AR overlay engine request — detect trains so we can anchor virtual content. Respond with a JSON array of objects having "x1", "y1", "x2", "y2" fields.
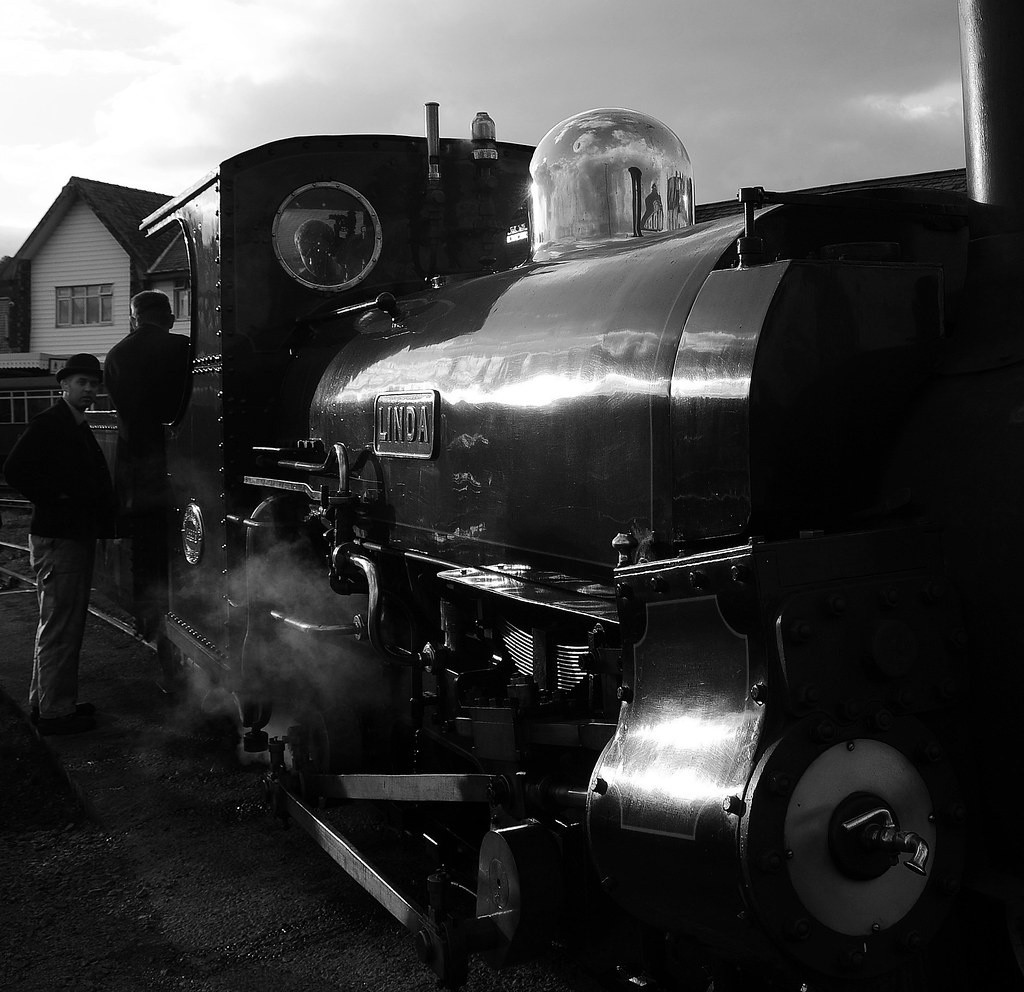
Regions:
[{"x1": 78, "y1": 102, "x2": 1024, "y2": 992}]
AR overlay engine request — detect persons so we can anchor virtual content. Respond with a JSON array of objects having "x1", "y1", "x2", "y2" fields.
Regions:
[
  {"x1": 0, "y1": 353, "x2": 119, "y2": 731},
  {"x1": 102, "y1": 290, "x2": 192, "y2": 641}
]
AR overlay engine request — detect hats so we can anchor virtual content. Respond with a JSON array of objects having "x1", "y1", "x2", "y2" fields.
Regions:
[{"x1": 56, "y1": 354, "x2": 104, "y2": 382}]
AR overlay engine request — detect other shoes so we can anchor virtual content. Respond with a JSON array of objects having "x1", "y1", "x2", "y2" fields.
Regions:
[{"x1": 31, "y1": 704, "x2": 96, "y2": 736}]
